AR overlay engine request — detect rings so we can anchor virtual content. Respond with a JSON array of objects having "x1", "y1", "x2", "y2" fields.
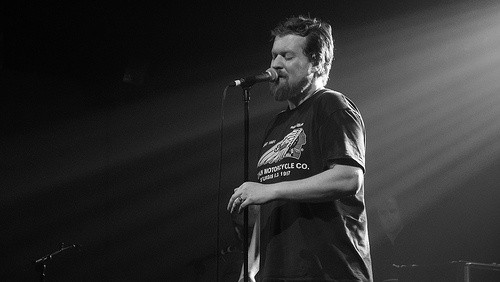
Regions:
[{"x1": 238, "y1": 196, "x2": 244, "y2": 204}]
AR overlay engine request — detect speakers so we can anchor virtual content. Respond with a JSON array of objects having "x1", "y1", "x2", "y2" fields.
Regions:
[{"x1": 456, "y1": 260, "x2": 500, "y2": 282}]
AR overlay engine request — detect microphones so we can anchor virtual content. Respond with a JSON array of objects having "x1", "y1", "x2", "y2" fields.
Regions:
[{"x1": 228, "y1": 67, "x2": 279, "y2": 88}]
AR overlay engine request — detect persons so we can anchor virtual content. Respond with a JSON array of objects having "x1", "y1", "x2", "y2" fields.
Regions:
[{"x1": 225, "y1": 15, "x2": 373, "y2": 282}]
